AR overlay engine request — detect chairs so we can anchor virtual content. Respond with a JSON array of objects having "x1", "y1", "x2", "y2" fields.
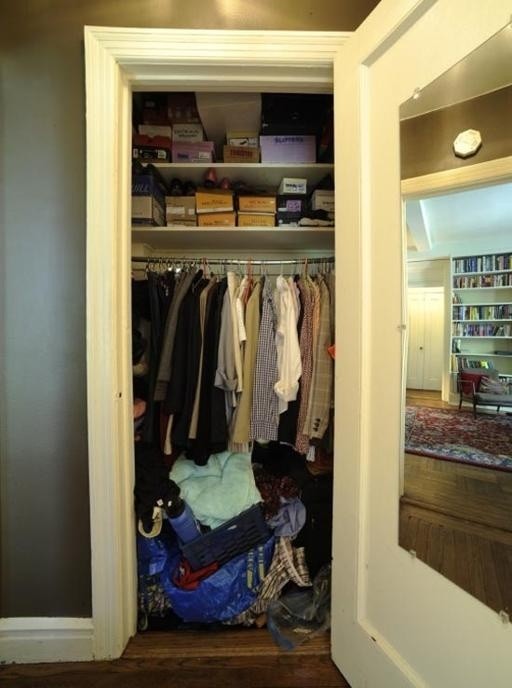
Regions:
[{"x1": 455, "y1": 367, "x2": 509, "y2": 418}]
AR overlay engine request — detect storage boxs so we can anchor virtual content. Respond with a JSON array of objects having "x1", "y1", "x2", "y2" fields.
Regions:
[{"x1": 131, "y1": 118, "x2": 335, "y2": 228}]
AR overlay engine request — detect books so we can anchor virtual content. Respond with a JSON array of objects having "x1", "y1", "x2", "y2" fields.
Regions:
[{"x1": 451, "y1": 256, "x2": 512, "y2": 371}]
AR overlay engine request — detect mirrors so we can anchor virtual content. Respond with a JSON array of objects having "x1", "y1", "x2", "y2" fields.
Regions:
[{"x1": 396, "y1": 151, "x2": 511, "y2": 626}]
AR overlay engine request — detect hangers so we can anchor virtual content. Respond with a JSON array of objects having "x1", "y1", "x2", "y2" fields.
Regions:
[{"x1": 132, "y1": 253, "x2": 334, "y2": 290}]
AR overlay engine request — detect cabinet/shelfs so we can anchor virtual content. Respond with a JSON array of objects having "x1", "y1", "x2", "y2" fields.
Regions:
[
  {"x1": 125, "y1": 84, "x2": 336, "y2": 247},
  {"x1": 444, "y1": 250, "x2": 511, "y2": 426}
]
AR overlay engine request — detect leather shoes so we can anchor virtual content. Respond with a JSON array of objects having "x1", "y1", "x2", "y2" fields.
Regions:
[{"x1": 132, "y1": 161, "x2": 249, "y2": 196}]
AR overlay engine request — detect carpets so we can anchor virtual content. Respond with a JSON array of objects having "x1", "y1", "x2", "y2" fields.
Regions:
[{"x1": 405, "y1": 402, "x2": 511, "y2": 471}]
